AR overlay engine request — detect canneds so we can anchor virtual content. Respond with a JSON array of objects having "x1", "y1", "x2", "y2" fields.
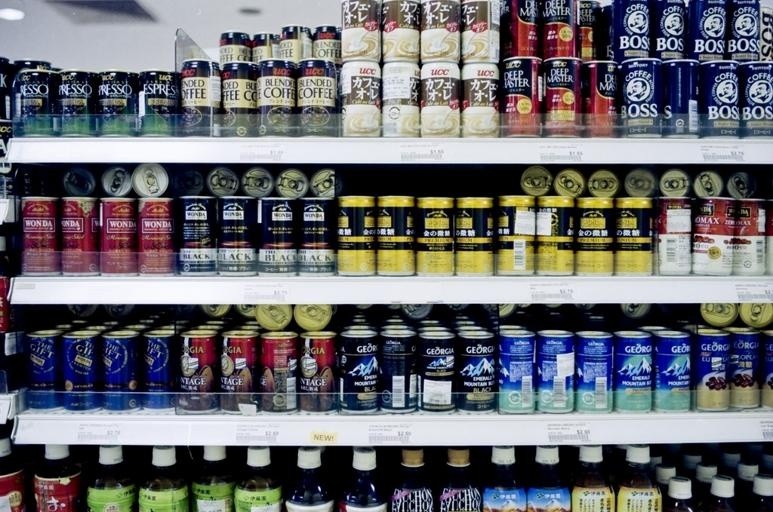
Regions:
[
  {"x1": 2, "y1": 303, "x2": 772, "y2": 418},
  {"x1": 0, "y1": 0, "x2": 772, "y2": 137},
  {"x1": 2, "y1": 165, "x2": 772, "y2": 275}
]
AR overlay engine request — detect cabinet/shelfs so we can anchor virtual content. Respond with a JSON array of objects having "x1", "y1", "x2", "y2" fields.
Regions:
[{"x1": 3, "y1": 25, "x2": 773, "y2": 455}]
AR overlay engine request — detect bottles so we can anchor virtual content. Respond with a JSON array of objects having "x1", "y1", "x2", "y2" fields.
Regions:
[
  {"x1": 0, "y1": 435, "x2": 773, "y2": 511},
  {"x1": 0, "y1": 236, "x2": 11, "y2": 334}
]
